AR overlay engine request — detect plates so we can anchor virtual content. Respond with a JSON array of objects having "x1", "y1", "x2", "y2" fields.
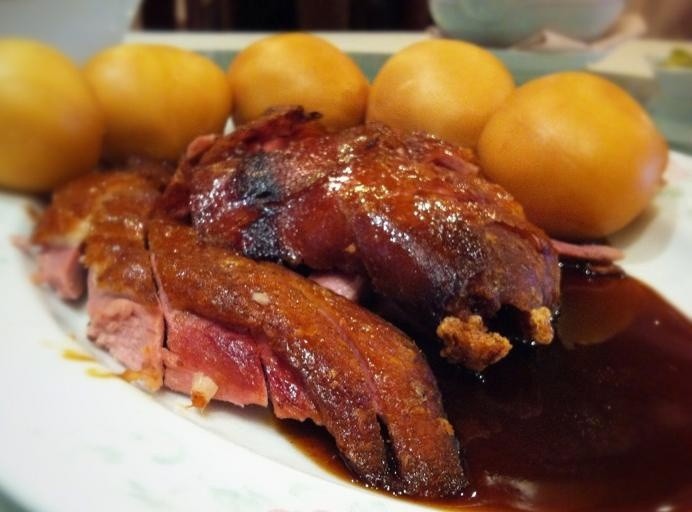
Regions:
[
  {"x1": 645, "y1": 103, "x2": 692, "y2": 146},
  {"x1": 0, "y1": 149, "x2": 692, "y2": 512},
  {"x1": 422, "y1": 30, "x2": 619, "y2": 85}
]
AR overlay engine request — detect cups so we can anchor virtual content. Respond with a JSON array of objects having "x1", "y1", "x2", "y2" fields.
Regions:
[
  {"x1": 0, "y1": 0, "x2": 139, "y2": 70},
  {"x1": 653, "y1": 69, "x2": 692, "y2": 125},
  {"x1": 428, "y1": 0, "x2": 621, "y2": 48}
]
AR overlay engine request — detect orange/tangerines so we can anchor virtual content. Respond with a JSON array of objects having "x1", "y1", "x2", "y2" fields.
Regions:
[
  {"x1": 476, "y1": 72, "x2": 669, "y2": 237},
  {"x1": 365, "y1": 39, "x2": 515, "y2": 149},
  {"x1": 226, "y1": 33, "x2": 371, "y2": 135},
  {"x1": 79, "y1": 43, "x2": 233, "y2": 166},
  {"x1": 0, "y1": 37, "x2": 106, "y2": 195}
]
